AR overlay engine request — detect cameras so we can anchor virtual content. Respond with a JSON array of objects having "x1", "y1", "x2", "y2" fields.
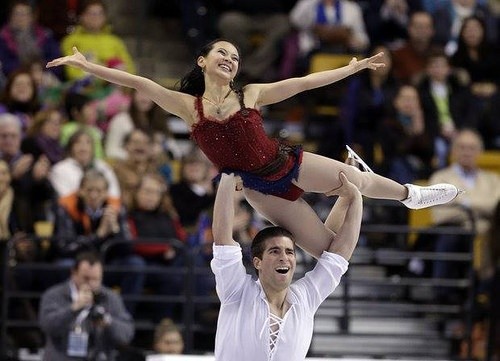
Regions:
[{"x1": 93, "y1": 292, "x2": 107, "y2": 305}]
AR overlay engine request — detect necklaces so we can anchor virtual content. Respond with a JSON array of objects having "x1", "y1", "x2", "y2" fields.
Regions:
[{"x1": 202, "y1": 90, "x2": 231, "y2": 115}]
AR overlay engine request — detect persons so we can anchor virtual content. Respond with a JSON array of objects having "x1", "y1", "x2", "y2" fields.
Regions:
[
  {"x1": 0, "y1": 0, "x2": 264, "y2": 361},
  {"x1": 219, "y1": 0, "x2": 500, "y2": 361},
  {"x1": 211, "y1": 172, "x2": 363, "y2": 361},
  {"x1": 46, "y1": 39, "x2": 458, "y2": 260}
]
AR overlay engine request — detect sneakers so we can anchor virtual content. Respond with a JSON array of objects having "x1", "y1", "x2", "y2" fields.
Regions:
[{"x1": 402, "y1": 183, "x2": 464, "y2": 209}]
